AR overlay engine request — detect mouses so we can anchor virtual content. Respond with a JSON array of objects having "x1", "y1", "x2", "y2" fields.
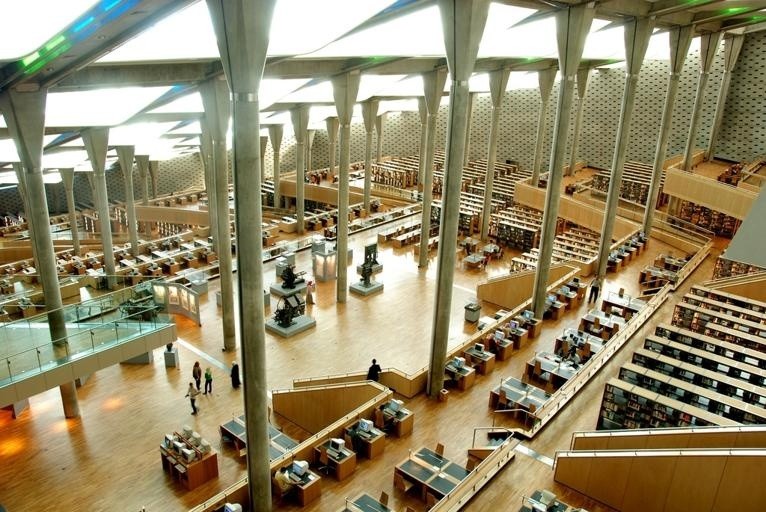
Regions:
[{"x1": 456, "y1": 370, "x2": 459, "y2": 372}]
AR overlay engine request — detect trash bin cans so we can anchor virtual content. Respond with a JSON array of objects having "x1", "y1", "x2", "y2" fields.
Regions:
[
  {"x1": 440, "y1": 389, "x2": 449, "y2": 402},
  {"x1": 222, "y1": 434, "x2": 234, "y2": 449},
  {"x1": 464, "y1": 303, "x2": 482, "y2": 323}
]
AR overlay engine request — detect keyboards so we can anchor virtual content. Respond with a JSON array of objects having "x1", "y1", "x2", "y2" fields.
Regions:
[
  {"x1": 174, "y1": 464, "x2": 186, "y2": 472},
  {"x1": 447, "y1": 365, "x2": 457, "y2": 371},
  {"x1": 290, "y1": 473, "x2": 302, "y2": 482}
]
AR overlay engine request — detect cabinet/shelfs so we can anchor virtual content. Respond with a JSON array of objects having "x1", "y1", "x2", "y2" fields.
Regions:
[
  {"x1": 517, "y1": 284, "x2": 766, "y2": 511},
  {"x1": 3, "y1": 188, "x2": 211, "y2": 239},
  {"x1": 227, "y1": 166, "x2": 333, "y2": 212},
  {"x1": 261, "y1": 241, "x2": 384, "y2": 339},
  {"x1": 75, "y1": 347, "x2": 180, "y2": 388},
  {"x1": 370, "y1": 149, "x2": 766, "y2": 282}
]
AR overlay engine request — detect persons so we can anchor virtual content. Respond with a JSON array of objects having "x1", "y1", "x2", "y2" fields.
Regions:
[
  {"x1": 366, "y1": 358, "x2": 381, "y2": 382},
  {"x1": 3, "y1": 214, "x2": 26, "y2": 227},
  {"x1": 465, "y1": 243, "x2": 470, "y2": 256},
  {"x1": 561, "y1": 349, "x2": 582, "y2": 366},
  {"x1": 192, "y1": 361, "x2": 202, "y2": 390},
  {"x1": 202, "y1": 367, "x2": 212, "y2": 394},
  {"x1": 184, "y1": 382, "x2": 201, "y2": 415},
  {"x1": 305, "y1": 169, "x2": 328, "y2": 186},
  {"x1": 587, "y1": 275, "x2": 602, "y2": 304},
  {"x1": 229, "y1": 360, "x2": 241, "y2": 390}
]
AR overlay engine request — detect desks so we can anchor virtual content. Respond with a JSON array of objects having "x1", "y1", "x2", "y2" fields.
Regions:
[
  {"x1": 1, "y1": 227, "x2": 236, "y2": 322},
  {"x1": 159, "y1": 428, "x2": 219, "y2": 491},
  {"x1": 220, "y1": 320, "x2": 554, "y2": 509},
  {"x1": 250, "y1": 196, "x2": 370, "y2": 260}
]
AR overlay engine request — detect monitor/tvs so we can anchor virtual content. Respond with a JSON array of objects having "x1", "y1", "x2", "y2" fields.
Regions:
[
  {"x1": 292, "y1": 398, "x2": 404, "y2": 475},
  {"x1": 452, "y1": 233, "x2": 645, "y2": 369},
  {"x1": 540, "y1": 489, "x2": 557, "y2": 509},
  {"x1": 224, "y1": 502, "x2": 242, "y2": 512},
  {"x1": 165, "y1": 425, "x2": 211, "y2": 464}
]
